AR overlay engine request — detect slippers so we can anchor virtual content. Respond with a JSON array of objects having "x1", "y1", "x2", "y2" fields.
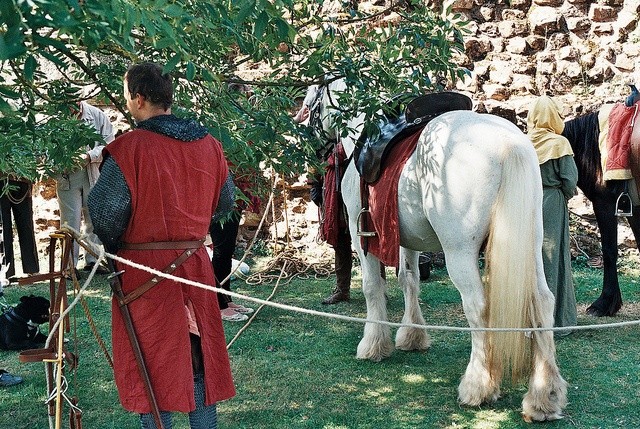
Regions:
[
  {"x1": 221, "y1": 308, "x2": 250, "y2": 322},
  {"x1": 235, "y1": 304, "x2": 255, "y2": 315}
]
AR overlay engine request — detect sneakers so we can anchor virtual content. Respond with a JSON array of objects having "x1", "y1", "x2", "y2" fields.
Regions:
[
  {"x1": 61, "y1": 268, "x2": 82, "y2": 281},
  {"x1": 324, "y1": 290, "x2": 351, "y2": 304},
  {"x1": 85, "y1": 262, "x2": 110, "y2": 274},
  {"x1": 385, "y1": 293, "x2": 390, "y2": 303}
]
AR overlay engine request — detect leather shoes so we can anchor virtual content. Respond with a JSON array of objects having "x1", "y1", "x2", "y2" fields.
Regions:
[
  {"x1": 0, "y1": 370, "x2": 24, "y2": 387},
  {"x1": 28, "y1": 272, "x2": 42, "y2": 277}
]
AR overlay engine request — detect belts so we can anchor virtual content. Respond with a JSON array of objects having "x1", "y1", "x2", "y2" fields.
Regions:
[
  {"x1": 114, "y1": 239, "x2": 205, "y2": 308},
  {"x1": 123, "y1": 239, "x2": 202, "y2": 250}
]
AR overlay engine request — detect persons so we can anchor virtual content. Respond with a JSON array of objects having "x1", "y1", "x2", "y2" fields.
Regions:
[
  {"x1": 210, "y1": 76, "x2": 309, "y2": 323},
  {"x1": 0, "y1": 92, "x2": 42, "y2": 281},
  {"x1": 54, "y1": 89, "x2": 114, "y2": 278},
  {"x1": 523, "y1": 95, "x2": 579, "y2": 336},
  {"x1": 310, "y1": 69, "x2": 379, "y2": 306},
  {"x1": 87, "y1": 61, "x2": 240, "y2": 429}
]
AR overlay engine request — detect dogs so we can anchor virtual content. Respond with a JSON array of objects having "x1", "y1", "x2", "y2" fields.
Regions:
[{"x1": 0, "y1": 293, "x2": 51, "y2": 350}]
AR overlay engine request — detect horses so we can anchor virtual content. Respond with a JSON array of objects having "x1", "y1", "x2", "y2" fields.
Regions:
[
  {"x1": 560, "y1": 109, "x2": 640, "y2": 316},
  {"x1": 304, "y1": 69, "x2": 571, "y2": 422}
]
